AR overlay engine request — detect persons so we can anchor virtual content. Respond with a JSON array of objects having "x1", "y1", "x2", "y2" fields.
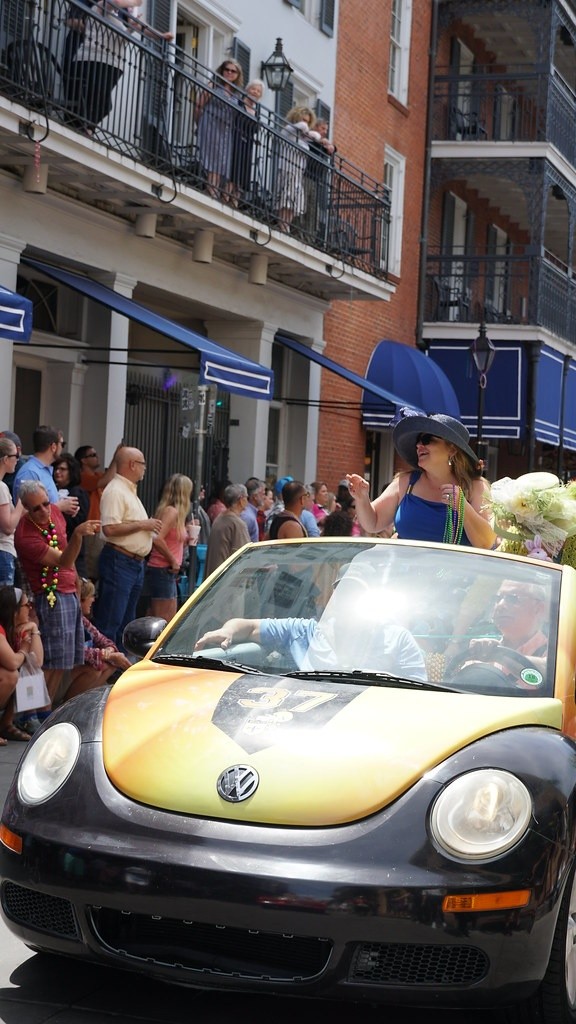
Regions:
[
  {"x1": 460, "y1": 578, "x2": 549, "y2": 689},
  {"x1": 271, "y1": 105, "x2": 337, "y2": 241},
  {"x1": 192, "y1": 59, "x2": 245, "y2": 201},
  {"x1": 0, "y1": 422, "x2": 162, "y2": 747},
  {"x1": 479, "y1": 470, "x2": 576, "y2": 570},
  {"x1": 61, "y1": 0, "x2": 174, "y2": 136},
  {"x1": 346, "y1": 414, "x2": 498, "y2": 574},
  {"x1": 194, "y1": 561, "x2": 429, "y2": 683},
  {"x1": 144, "y1": 468, "x2": 398, "y2": 634},
  {"x1": 221, "y1": 79, "x2": 263, "y2": 208}
]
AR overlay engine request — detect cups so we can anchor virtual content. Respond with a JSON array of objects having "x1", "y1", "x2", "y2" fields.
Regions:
[{"x1": 187, "y1": 525, "x2": 201, "y2": 546}]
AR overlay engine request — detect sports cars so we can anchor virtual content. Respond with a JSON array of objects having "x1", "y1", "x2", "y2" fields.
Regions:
[{"x1": 0, "y1": 536, "x2": 576, "y2": 1024}]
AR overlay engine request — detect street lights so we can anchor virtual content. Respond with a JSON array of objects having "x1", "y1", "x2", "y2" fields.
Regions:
[{"x1": 467, "y1": 325, "x2": 496, "y2": 460}]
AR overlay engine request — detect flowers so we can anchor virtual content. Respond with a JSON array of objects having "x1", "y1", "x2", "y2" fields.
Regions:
[{"x1": 478, "y1": 478, "x2": 576, "y2": 542}]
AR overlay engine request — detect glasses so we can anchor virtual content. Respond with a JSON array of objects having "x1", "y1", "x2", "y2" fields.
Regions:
[
  {"x1": 83, "y1": 453, "x2": 97, "y2": 458},
  {"x1": 81, "y1": 577, "x2": 88, "y2": 586},
  {"x1": 26, "y1": 501, "x2": 51, "y2": 513},
  {"x1": 20, "y1": 602, "x2": 31, "y2": 609},
  {"x1": 50, "y1": 439, "x2": 66, "y2": 448},
  {"x1": 223, "y1": 67, "x2": 238, "y2": 73},
  {"x1": 0, "y1": 452, "x2": 20, "y2": 460},
  {"x1": 200, "y1": 486, "x2": 205, "y2": 492},
  {"x1": 300, "y1": 493, "x2": 310, "y2": 502},
  {"x1": 415, "y1": 434, "x2": 440, "y2": 447},
  {"x1": 350, "y1": 505, "x2": 355, "y2": 509},
  {"x1": 54, "y1": 467, "x2": 69, "y2": 472},
  {"x1": 495, "y1": 593, "x2": 540, "y2": 607},
  {"x1": 131, "y1": 459, "x2": 147, "y2": 466}
]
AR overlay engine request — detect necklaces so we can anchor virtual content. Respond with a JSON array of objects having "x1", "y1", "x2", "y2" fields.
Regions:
[
  {"x1": 442, "y1": 484, "x2": 466, "y2": 544},
  {"x1": 27, "y1": 513, "x2": 59, "y2": 608},
  {"x1": 426, "y1": 652, "x2": 446, "y2": 683}
]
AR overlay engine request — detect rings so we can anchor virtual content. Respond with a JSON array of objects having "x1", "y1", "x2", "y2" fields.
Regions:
[{"x1": 446, "y1": 495, "x2": 449, "y2": 500}]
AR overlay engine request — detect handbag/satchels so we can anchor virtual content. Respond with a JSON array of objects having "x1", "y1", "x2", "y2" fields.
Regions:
[{"x1": 13, "y1": 651, "x2": 46, "y2": 713}]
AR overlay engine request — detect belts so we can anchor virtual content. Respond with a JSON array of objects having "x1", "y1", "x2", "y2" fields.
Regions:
[{"x1": 107, "y1": 541, "x2": 145, "y2": 563}]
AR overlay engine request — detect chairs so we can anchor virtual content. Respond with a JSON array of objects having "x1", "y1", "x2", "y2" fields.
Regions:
[
  {"x1": 149, "y1": 124, "x2": 207, "y2": 192},
  {"x1": 487, "y1": 304, "x2": 530, "y2": 326},
  {"x1": 451, "y1": 104, "x2": 488, "y2": 141},
  {"x1": 7, "y1": 39, "x2": 87, "y2": 132},
  {"x1": 239, "y1": 181, "x2": 376, "y2": 274},
  {"x1": 432, "y1": 277, "x2": 471, "y2": 322}
]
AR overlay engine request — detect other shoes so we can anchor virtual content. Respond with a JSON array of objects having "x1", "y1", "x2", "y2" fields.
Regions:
[
  {"x1": 26, "y1": 718, "x2": 42, "y2": 734},
  {"x1": 0, "y1": 722, "x2": 31, "y2": 745}
]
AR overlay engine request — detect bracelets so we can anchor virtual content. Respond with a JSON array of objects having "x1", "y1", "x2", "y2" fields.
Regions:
[{"x1": 31, "y1": 631, "x2": 41, "y2": 635}]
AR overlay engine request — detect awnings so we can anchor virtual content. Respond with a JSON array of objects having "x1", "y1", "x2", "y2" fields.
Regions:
[
  {"x1": 0, "y1": 284, "x2": 33, "y2": 344},
  {"x1": 274, "y1": 334, "x2": 426, "y2": 429},
  {"x1": 13, "y1": 255, "x2": 275, "y2": 401},
  {"x1": 361, "y1": 338, "x2": 461, "y2": 429}
]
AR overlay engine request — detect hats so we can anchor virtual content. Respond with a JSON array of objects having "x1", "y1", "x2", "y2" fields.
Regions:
[
  {"x1": 392, "y1": 413, "x2": 484, "y2": 478},
  {"x1": 332, "y1": 561, "x2": 375, "y2": 591},
  {"x1": 491, "y1": 471, "x2": 576, "y2": 546},
  {"x1": 274, "y1": 476, "x2": 293, "y2": 494}
]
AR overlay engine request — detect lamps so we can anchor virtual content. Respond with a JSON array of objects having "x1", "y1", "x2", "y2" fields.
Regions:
[{"x1": 260, "y1": 37, "x2": 294, "y2": 91}]
